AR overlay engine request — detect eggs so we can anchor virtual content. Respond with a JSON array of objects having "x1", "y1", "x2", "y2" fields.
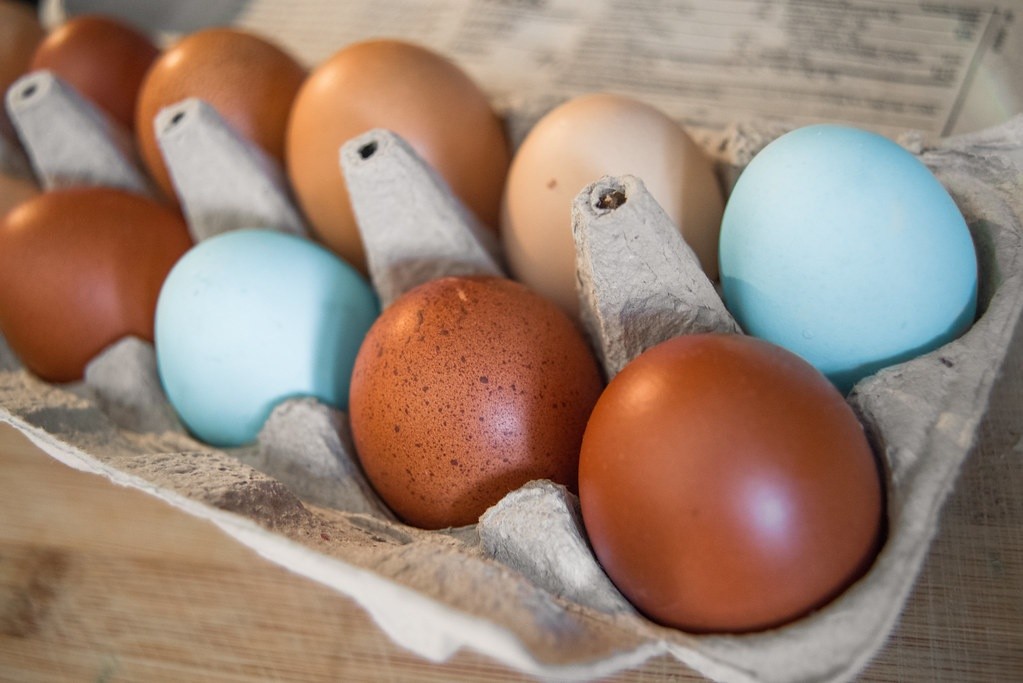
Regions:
[{"x1": 0, "y1": 7, "x2": 976, "y2": 634}]
